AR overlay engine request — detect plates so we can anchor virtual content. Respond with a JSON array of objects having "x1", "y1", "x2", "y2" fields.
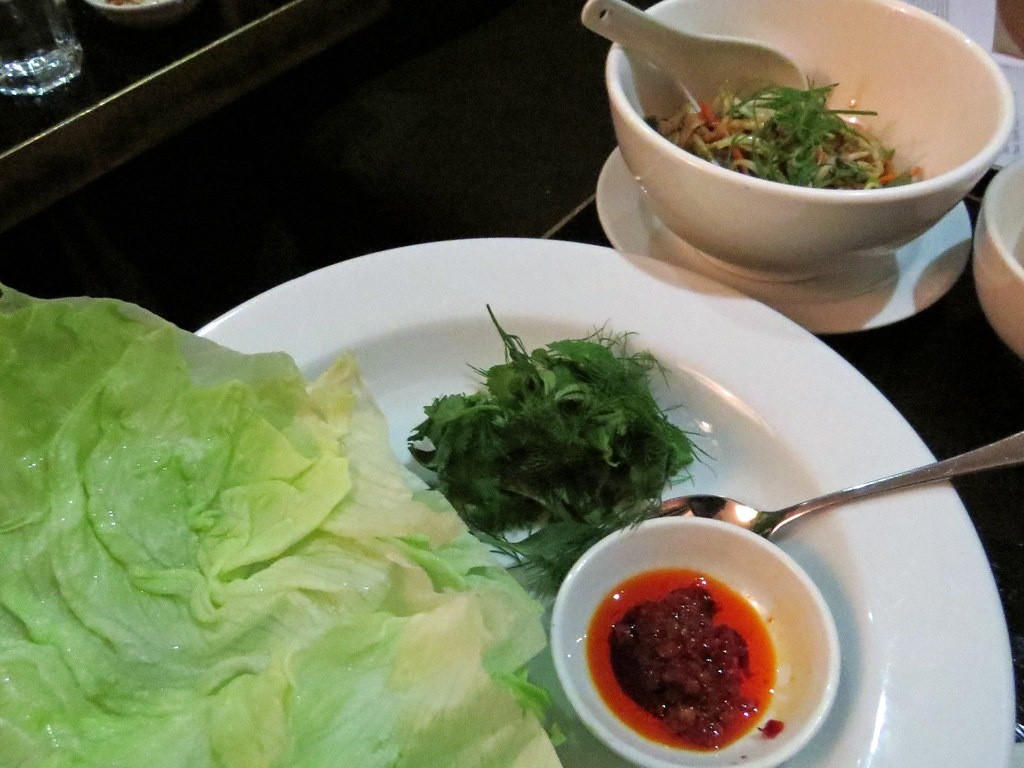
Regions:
[
  {"x1": 595, "y1": 145, "x2": 974, "y2": 333},
  {"x1": 195, "y1": 236, "x2": 1015, "y2": 768}
]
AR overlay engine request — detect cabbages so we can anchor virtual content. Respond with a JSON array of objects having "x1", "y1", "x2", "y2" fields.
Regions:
[{"x1": 0, "y1": 284, "x2": 578, "y2": 768}]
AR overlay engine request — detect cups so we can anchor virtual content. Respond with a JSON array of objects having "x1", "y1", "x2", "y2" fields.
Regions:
[{"x1": 0, "y1": 0, "x2": 84, "y2": 98}]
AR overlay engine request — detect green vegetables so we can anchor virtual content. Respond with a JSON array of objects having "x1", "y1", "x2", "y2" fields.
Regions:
[
  {"x1": 403, "y1": 297, "x2": 715, "y2": 600},
  {"x1": 707, "y1": 71, "x2": 914, "y2": 197}
]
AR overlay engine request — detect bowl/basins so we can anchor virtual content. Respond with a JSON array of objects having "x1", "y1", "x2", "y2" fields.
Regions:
[
  {"x1": 548, "y1": 515, "x2": 841, "y2": 768},
  {"x1": 605, "y1": 0, "x2": 1015, "y2": 283},
  {"x1": 973, "y1": 159, "x2": 1024, "y2": 362}
]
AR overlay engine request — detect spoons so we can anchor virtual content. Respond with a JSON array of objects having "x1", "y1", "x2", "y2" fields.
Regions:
[
  {"x1": 582, "y1": 0, "x2": 808, "y2": 116},
  {"x1": 650, "y1": 429, "x2": 1024, "y2": 541}
]
